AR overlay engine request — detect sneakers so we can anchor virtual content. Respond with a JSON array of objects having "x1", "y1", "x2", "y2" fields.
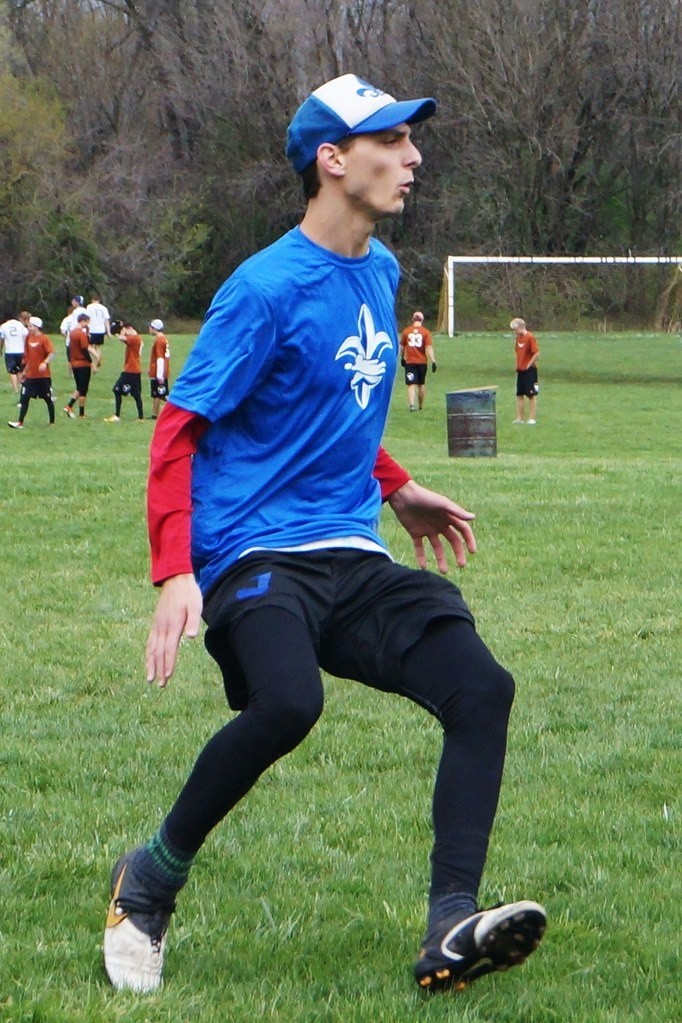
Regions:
[
  {"x1": 103, "y1": 848, "x2": 180, "y2": 998},
  {"x1": 414, "y1": 902, "x2": 548, "y2": 997}
]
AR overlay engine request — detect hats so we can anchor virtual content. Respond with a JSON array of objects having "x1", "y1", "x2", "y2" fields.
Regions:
[
  {"x1": 413, "y1": 312, "x2": 424, "y2": 322},
  {"x1": 29, "y1": 317, "x2": 43, "y2": 327},
  {"x1": 284, "y1": 74, "x2": 440, "y2": 175},
  {"x1": 146, "y1": 319, "x2": 163, "y2": 331},
  {"x1": 74, "y1": 296, "x2": 85, "y2": 307}
]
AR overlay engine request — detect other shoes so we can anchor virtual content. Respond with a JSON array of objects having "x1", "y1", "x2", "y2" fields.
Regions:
[
  {"x1": 9, "y1": 420, "x2": 23, "y2": 429},
  {"x1": 103, "y1": 414, "x2": 119, "y2": 423},
  {"x1": 136, "y1": 418, "x2": 145, "y2": 424},
  {"x1": 512, "y1": 419, "x2": 524, "y2": 424},
  {"x1": 63, "y1": 405, "x2": 75, "y2": 418},
  {"x1": 409, "y1": 406, "x2": 416, "y2": 413},
  {"x1": 526, "y1": 419, "x2": 536, "y2": 426},
  {"x1": 78, "y1": 413, "x2": 87, "y2": 419}
]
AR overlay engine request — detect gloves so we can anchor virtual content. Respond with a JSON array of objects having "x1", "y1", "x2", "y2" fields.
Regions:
[
  {"x1": 400, "y1": 358, "x2": 407, "y2": 368},
  {"x1": 431, "y1": 362, "x2": 437, "y2": 373}
]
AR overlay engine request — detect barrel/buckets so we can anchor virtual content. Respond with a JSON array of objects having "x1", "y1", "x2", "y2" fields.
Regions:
[{"x1": 445, "y1": 390, "x2": 497, "y2": 459}]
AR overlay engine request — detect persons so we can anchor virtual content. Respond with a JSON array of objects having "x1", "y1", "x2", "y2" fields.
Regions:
[
  {"x1": 103, "y1": 320, "x2": 145, "y2": 423},
  {"x1": 103, "y1": 73, "x2": 549, "y2": 996},
  {"x1": 146, "y1": 319, "x2": 169, "y2": 420},
  {"x1": 62, "y1": 314, "x2": 104, "y2": 419},
  {"x1": 510, "y1": 318, "x2": 539, "y2": 425},
  {"x1": 60, "y1": 294, "x2": 113, "y2": 377},
  {"x1": 0, "y1": 311, "x2": 57, "y2": 408},
  {"x1": 399, "y1": 311, "x2": 437, "y2": 412},
  {"x1": 7, "y1": 317, "x2": 56, "y2": 429}
]
